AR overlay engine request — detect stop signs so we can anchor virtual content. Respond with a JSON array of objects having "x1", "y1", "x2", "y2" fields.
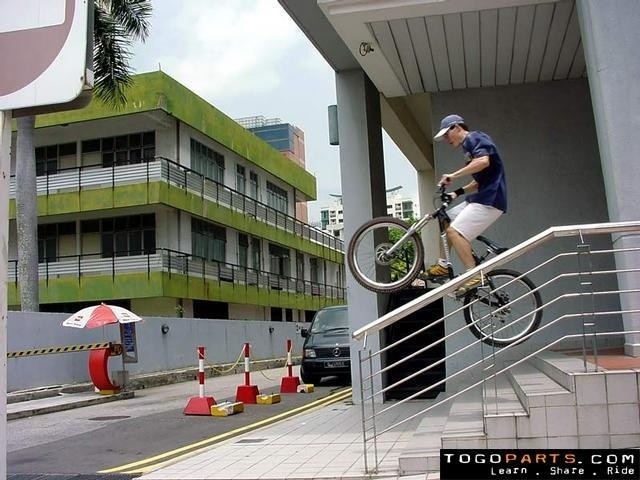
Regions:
[{"x1": 0, "y1": 0, "x2": 89, "y2": 110}]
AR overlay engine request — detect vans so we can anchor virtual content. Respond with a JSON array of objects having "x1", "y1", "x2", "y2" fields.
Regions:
[{"x1": 299, "y1": 306, "x2": 350, "y2": 386}]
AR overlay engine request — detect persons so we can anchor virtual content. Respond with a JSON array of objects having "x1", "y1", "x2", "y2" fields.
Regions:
[{"x1": 422, "y1": 114, "x2": 508, "y2": 296}]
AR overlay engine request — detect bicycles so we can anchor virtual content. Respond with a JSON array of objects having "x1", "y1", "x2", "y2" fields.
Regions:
[{"x1": 347, "y1": 175, "x2": 543, "y2": 349}]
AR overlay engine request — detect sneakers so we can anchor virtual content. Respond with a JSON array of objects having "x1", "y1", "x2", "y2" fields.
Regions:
[
  {"x1": 421, "y1": 263, "x2": 453, "y2": 281},
  {"x1": 456, "y1": 273, "x2": 489, "y2": 297}
]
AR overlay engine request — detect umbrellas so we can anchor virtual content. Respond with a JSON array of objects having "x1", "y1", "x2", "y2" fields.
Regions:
[{"x1": 63, "y1": 301, "x2": 143, "y2": 342}]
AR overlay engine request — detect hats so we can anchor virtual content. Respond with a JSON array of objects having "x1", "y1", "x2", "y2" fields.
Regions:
[{"x1": 433, "y1": 113, "x2": 464, "y2": 144}]
